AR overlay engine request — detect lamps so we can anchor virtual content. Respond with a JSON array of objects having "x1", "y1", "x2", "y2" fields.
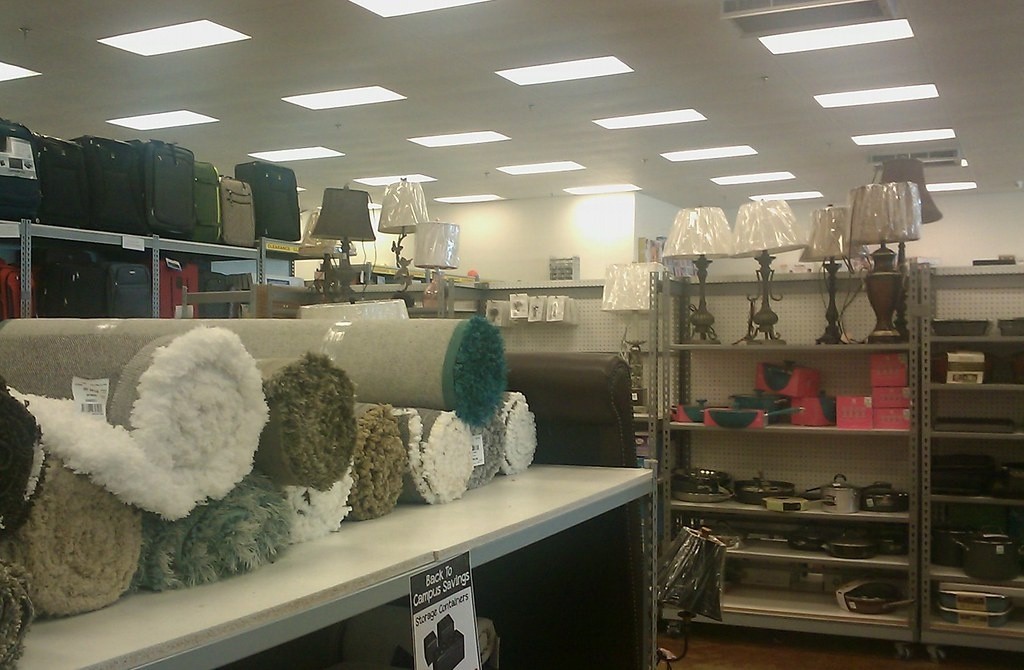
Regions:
[
  {"x1": 663, "y1": 158, "x2": 944, "y2": 346},
  {"x1": 298, "y1": 179, "x2": 460, "y2": 314}
]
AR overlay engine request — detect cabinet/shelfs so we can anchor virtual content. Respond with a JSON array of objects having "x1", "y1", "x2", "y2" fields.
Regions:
[
  {"x1": 0, "y1": 319, "x2": 657, "y2": 670},
  {"x1": 663, "y1": 258, "x2": 925, "y2": 659},
  {"x1": 919, "y1": 262, "x2": 1024, "y2": 668},
  {"x1": 1, "y1": 221, "x2": 458, "y2": 322},
  {"x1": 450, "y1": 271, "x2": 662, "y2": 625}
]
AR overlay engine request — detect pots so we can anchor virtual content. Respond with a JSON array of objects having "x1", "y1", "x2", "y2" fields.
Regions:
[
  {"x1": 930, "y1": 524, "x2": 1024, "y2": 582},
  {"x1": 931, "y1": 454, "x2": 1024, "y2": 500},
  {"x1": 931, "y1": 314, "x2": 1024, "y2": 336},
  {"x1": 672, "y1": 463, "x2": 909, "y2": 559},
  {"x1": 833, "y1": 584, "x2": 915, "y2": 615},
  {"x1": 683, "y1": 361, "x2": 835, "y2": 427}
]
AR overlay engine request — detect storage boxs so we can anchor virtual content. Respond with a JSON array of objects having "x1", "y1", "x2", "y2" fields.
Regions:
[{"x1": 674, "y1": 352, "x2": 911, "y2": 430}]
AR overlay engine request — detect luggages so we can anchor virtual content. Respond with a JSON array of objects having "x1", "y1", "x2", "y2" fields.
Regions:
[{"x1": 0, "y1": 118, "x2": 301, "y2": 249}]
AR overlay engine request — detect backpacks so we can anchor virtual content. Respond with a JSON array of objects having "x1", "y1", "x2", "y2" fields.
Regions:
[{"x1": 0, "y1": 240, "x2": 235, "y2": 318}]
